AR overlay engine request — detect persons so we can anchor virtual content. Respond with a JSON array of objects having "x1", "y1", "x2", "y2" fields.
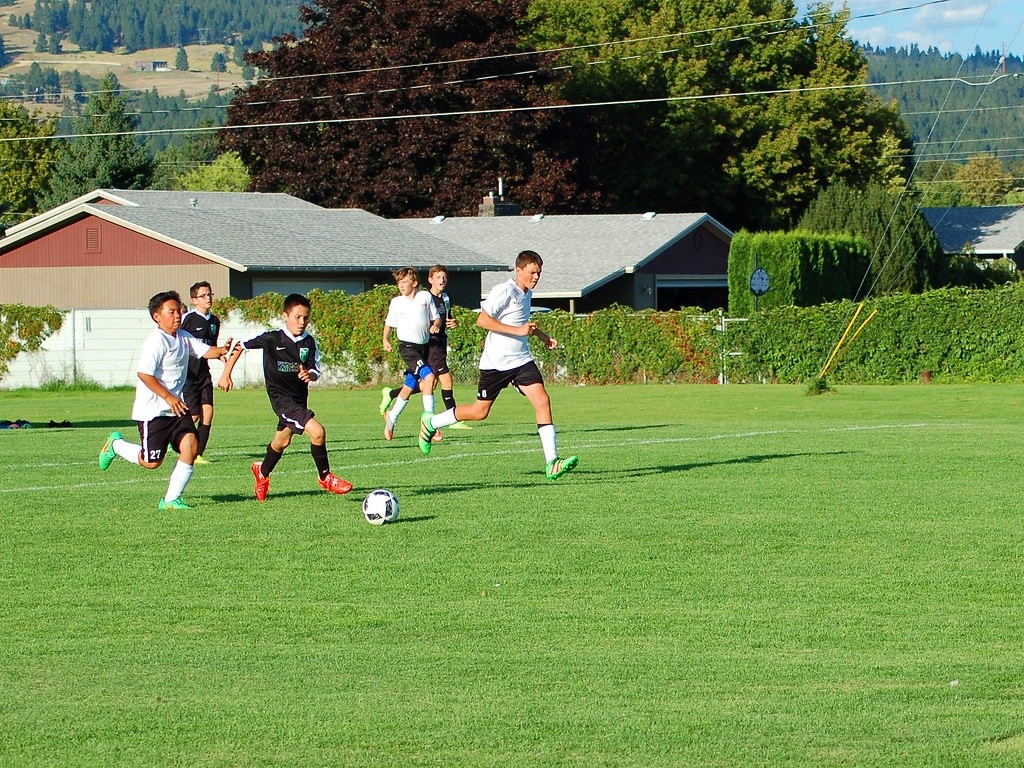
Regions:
[
  {"x1": 99, "y1": 280, "x2": 233, "y2": 510},
  {"x1": 378, "y1": 265, "x2": 473, "y2": 441},
  {"x1": 217, "y1": 293, "x2": 352, "y2": 501},
  {"x1": 416, "y1": 250, "x2": 578, "y2": 481}
]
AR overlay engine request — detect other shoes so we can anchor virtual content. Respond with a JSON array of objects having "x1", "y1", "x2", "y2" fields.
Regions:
[{"x1": 193, "y1": 454, "x2": 208, "y2": 464}]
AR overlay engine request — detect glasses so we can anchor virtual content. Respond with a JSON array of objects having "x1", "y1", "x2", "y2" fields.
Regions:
[{"x1": 198, "y1": 292, "x2": 214, "y2": 299}]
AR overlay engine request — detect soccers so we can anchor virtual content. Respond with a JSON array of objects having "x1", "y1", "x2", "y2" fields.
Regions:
[{"x1": 361, "y1": 489, "x2": 401, "y2": 526}]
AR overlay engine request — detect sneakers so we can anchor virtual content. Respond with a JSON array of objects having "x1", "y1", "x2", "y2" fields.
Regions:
[
  {"x1": 378, "y1": 386, "x2": 393, "y2": 416},
  {"x1": 99, "y1": 431, "x2": 123, "y2": 470},
  {"x1": 384, "y1": 411, "x2": 397, "y2": 440},
  {"x1": 317, "y1": 472, "x2": 353, "y2": 495},
  {"x1": 158, "y1": 497, "x2": 193, "y2": 510},
  {"x1": 251, "y1": 461, "x2": 270, "y2": 502},
  {"x1": 431, "y1": 429, "x2": 443, "y2": 442},
  {"x1": 418, "y1": 411, "x2": 436, "y2": 454},
  {"x1": 449, "y1": 422, "x2": 472, "y2": 430},
  {"x1": 545, "y1": 455, "x2": 579, "y2": 481}
]
